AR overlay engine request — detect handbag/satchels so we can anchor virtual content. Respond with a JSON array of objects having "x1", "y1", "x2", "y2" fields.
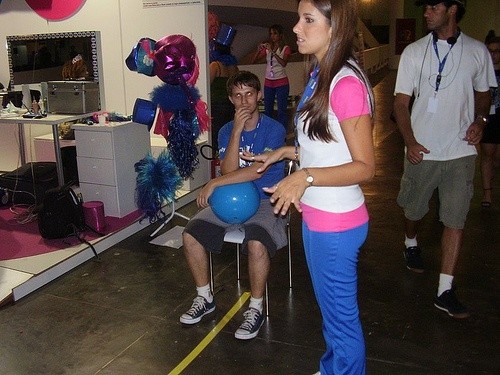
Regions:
[{"x1": 58, "y1": 117, "x2": 87, "y2": 139}]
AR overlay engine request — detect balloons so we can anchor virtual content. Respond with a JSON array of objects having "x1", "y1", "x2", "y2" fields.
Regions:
[{"x1": 209, "y1": 182, "x2": 260, "y2": 224}]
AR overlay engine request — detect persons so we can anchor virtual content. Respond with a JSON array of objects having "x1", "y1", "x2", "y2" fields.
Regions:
[
  {"x1": 180, "y1": 71, "x2": 291, "y2": 339},
  {"x1": 240, "y1": 0, "x2": 376, "y2": 375},
  {"x1": 474, "y1": 30, "x2": 500, "y2": 207},
  {"x1": 209, "y1": 22, "x2": 241, "y2": 160},
  {"x1": 394, "y1": 1, "x2": 498, "y2": 317},
  {"x1": 253, "y1": 24, "x2": 291, "y2": 143}
]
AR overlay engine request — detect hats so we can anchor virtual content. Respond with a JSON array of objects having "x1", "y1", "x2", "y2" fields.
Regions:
[
  {"x1": 132, "y1": 97, "x2": 161, "y2": 133},
  {"x1": 213, "y1": 21, "x2": 237, "y2": 49}
]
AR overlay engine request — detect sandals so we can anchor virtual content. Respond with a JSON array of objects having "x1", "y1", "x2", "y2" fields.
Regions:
[{"x1": 481, "y1": 188, "x2": 492, "y2": 207}]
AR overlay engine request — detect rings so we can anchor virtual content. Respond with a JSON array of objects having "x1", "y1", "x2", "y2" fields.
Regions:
[{"x1": 252, "y1": 157, "x2": 255, "y2": 161}]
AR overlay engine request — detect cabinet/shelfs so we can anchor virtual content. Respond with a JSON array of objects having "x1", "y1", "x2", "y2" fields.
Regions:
[{"x1": 72, "y1": 117, "x2": 150, "y2": 217}]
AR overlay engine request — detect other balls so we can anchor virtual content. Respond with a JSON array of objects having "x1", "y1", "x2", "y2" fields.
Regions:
[{"x1": 209, "y1": 181, "x2": 261, "y2": 225}]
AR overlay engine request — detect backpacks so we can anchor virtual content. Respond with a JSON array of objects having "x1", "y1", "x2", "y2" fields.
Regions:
[{"x1": 39, "y1": 186, "x2": 84, "y2": 238}]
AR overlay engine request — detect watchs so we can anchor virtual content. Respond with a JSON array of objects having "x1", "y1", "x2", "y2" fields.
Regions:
[
  {"x1": 302, "y1": 168, "x2": 313, "y2": 186},
  {"x1": 475, "y1": 114, "x2": 488, "y2": 124}
]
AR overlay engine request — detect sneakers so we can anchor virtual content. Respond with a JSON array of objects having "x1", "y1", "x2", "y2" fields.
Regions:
[
  {"x1": 235, "y1": 307, "x2": 266, "y2": 339},
  {"x1": 180, "y1": 295, "x2": 215, "y2": 324},
  {"x1": 433, "y1": 288, "x2": 469, "y2": 318},
  {"x1": 403, "y1": 244, "x2": 424, "y2": 273}
]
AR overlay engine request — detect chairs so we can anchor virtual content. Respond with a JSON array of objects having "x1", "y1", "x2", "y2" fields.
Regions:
[{"x1": 210, "y1": 159, "x2": 294, "y2": 316}]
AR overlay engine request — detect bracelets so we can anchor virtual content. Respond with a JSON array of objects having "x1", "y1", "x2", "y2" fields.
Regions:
[
  {"x1": 295, "y1": 146, "x2": 298, "y2": 161},
  {"x1": 272, "y1": 53, "x2": 276, "y2": 55}
]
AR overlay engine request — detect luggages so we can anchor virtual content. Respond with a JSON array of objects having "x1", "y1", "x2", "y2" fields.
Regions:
[{"x1": 0, "y1": 161, "x2": 79, "y2": 208}]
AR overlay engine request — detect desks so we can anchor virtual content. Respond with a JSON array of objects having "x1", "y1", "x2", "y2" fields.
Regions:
[{"x1": 0, "y1": 109, "x2": 103, "y2": 188}]
